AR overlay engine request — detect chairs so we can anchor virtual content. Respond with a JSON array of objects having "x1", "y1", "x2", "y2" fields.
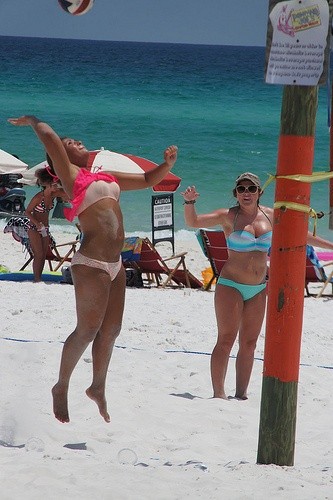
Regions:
[
  {"x1": 304, "y1": 245, "x2": 333, "y2": 299},
  {"x1": 122, "y1": 236, "x2": 202, "y2": 288},
  {"x1": 3, "y1": 216, "x2": 78, "y2": 271},
  {"x1": 199, "y1": 229, "x2": 228, "y2": 290}
]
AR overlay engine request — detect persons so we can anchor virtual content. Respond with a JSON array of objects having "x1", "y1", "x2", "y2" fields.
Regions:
[
  {"x1": 8, "y1": 114, "x2": 178, "y2": 423},
  {"x1": 24, "y1": 168, "x2": 69, "y2": 282},
  {"x1": 182, "y1": 172, "x2": 333, "y2": 400}
]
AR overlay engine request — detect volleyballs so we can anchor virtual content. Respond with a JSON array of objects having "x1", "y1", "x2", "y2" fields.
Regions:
[{"x1": 56, "y1": 0, "x2": 95, "y2": 16}]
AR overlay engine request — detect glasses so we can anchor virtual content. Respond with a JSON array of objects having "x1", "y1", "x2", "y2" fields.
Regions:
[{"x1": 235, "y1": 184, "x2": 259, "y2": 195}]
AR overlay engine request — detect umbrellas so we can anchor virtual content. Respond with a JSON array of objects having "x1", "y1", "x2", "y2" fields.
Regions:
[
  {"x1": 21, "y1": 148, "x2": 182, "y2": 193},
  {"x1": 0, "y1": 149, "x2": 28, "y2": 174}
]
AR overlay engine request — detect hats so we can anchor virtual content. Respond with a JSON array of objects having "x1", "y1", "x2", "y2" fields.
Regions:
[{"x1": 235, "y1": 172, "x2": 261, "y2": 188}]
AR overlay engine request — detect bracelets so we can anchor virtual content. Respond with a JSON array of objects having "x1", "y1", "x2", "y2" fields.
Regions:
[{"x1": 185, "y1": 200, "x2": 196, "y2": 204}]
